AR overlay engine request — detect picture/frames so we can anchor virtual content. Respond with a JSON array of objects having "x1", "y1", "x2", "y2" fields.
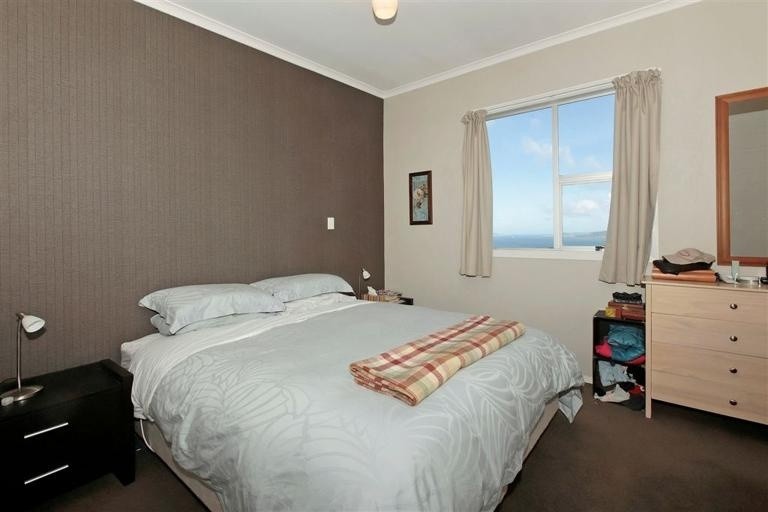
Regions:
[{"x1": 408, "y1": 171, "x2": 433, "y2": 225}]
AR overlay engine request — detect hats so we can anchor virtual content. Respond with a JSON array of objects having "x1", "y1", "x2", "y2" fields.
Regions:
[{"x1": 662, "y1": 249, "x2": 716, "y2": 265}]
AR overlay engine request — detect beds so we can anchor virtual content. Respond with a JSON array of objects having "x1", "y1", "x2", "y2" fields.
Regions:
[{"x1": 120, "y1": 300, "x2": 586, "y2": 512}]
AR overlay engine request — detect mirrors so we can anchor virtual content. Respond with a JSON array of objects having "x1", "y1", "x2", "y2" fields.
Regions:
[{"x1": 715, "y1": 88, "x2": 768, "y2": 267}]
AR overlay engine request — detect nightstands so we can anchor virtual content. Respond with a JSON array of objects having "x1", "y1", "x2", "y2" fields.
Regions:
[
  {"x1": 397, "y1": 296, "x2": 413, "y2": 305},
  {"x1": 0, "y1": 359, "x2": 134, "y2": 512}
]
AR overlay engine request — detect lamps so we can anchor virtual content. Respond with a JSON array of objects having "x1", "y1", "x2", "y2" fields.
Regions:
[
  {"x1": 359, "y1": 267, "x2": 370, "y2": 300},
  {"x1": 372, "y1": 0, "x2": 398, "y2": 19},
  {"x1": 0, "y1": 312, "x2": 46, "y2": 401}
]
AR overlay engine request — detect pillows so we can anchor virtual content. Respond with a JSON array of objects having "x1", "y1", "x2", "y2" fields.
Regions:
[
  {"x1": 249, "y1": 272, "x2": 355, "y2": 303},
  {"x1": 286, "y1": 292, "x2": 357, "y2": 312},
  {"x1": 151, "y1": 312, "x2": 281, "y2": 335},
  {"x1": 139, "y1": 283, "x2": 286, "y2": 335}
]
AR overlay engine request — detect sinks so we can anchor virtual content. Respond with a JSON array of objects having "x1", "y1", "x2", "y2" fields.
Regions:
[{"x1": 728, "y1": 275, "x2": 761, "y2": 285}]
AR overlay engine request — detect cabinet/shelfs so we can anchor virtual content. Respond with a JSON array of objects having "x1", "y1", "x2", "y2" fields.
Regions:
[
  {"x1": 592, "y1": 311, "x2": 645, "y2": 395},
  {"x1": 640, "y1": 275, "x2": 768, "y2": 425}
]
